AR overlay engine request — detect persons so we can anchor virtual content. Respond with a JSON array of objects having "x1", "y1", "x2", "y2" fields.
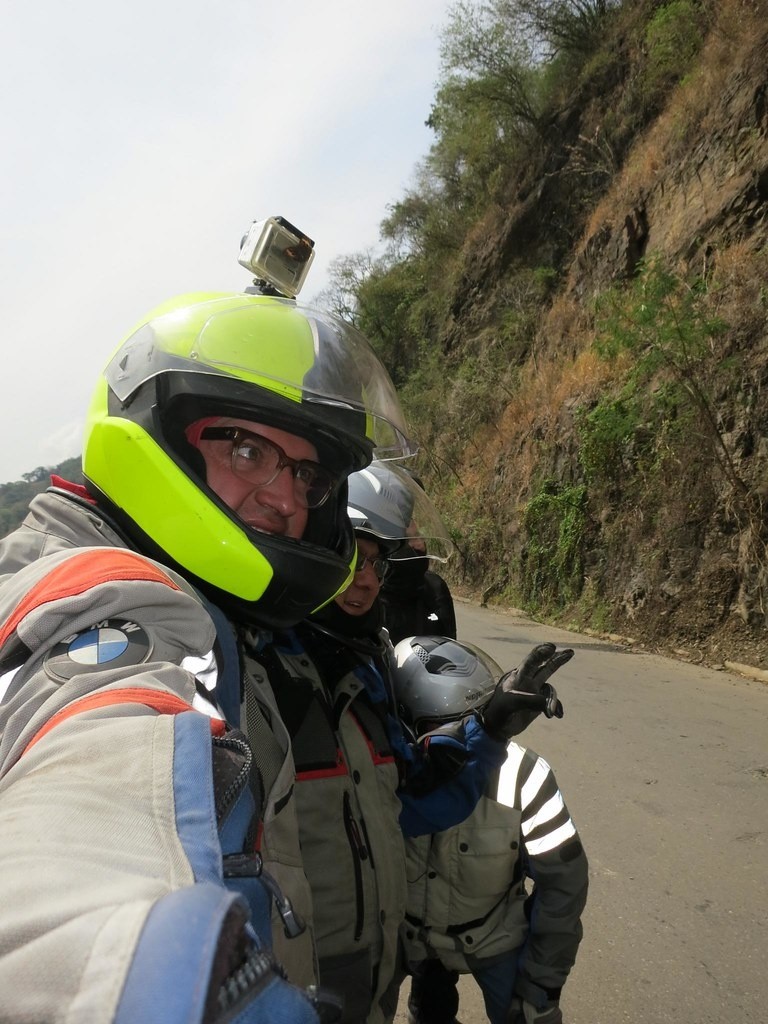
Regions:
[
  {"x1": 379, "y1": 532, "x2": 455, "y2": 646},
  {"x1": 244, "y1": 451, "x2": 452, "y2": 1024},
  {"x1": 394, "y1": 636, "x2": 589, "y2": 1024},
  {"x1": 0, "y1": 212, "x2": 419, "y2": 1024}
]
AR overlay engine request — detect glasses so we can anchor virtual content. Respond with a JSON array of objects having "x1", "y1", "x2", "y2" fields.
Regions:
[
  {"x1": 199, "y1": 426, "x2": 341, "y2": 510},
  {"x1": 355, "y1": 550, "x2": 392, "y2": 578}
]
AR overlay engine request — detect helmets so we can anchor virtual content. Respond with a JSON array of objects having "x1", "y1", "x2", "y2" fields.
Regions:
[
  {"x1": 391, "y1": 635, "x2": 504, "y2": 744},
  {"x1": 81, "y1": 292, "x2": 378, "y2": 634},
  {"x1": 303, "y1": 466, "x2": 406, "y2": 655}
]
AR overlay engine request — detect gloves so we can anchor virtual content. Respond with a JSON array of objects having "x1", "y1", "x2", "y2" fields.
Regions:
[
  {"x1": 506, "y1": 997, "x2": 562, "y2": 1024},
  {"x1": 474, "y1": 643, "x2": 575, "y2": 744}
]
